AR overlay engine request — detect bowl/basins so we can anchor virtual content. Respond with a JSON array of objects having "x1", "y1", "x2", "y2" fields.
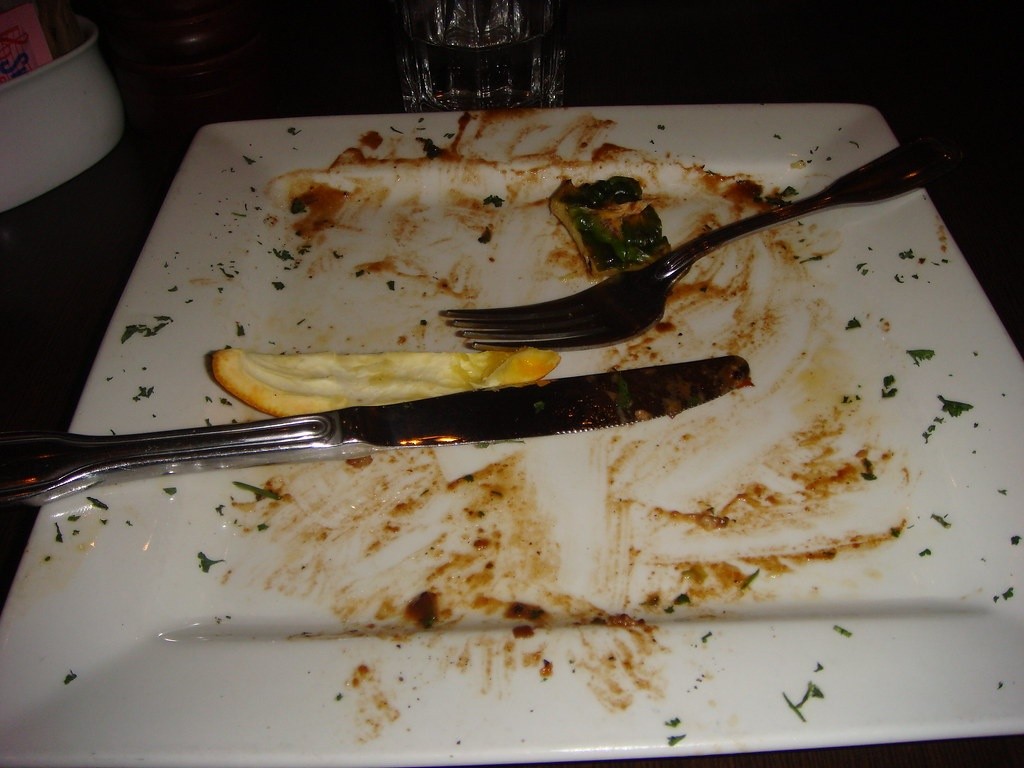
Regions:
[{"x1": 0, "y1": 17, "x2": 124, "y2": 214}]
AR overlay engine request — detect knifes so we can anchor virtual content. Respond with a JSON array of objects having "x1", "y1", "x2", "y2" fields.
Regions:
[{"x1": 0, "y1": 355, "x2": 750, "y2": 505}]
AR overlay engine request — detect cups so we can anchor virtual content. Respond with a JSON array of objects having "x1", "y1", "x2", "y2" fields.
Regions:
[{"x1": 396, "y1": 0, "x2": 565, "y2": 111}]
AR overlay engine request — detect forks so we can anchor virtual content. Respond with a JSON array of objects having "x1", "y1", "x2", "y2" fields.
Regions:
[{"x1": 445, "y1": 130, "x2": 965, "y2": 350}]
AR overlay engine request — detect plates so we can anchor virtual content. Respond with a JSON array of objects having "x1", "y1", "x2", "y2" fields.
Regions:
[{"x1": 0, "y1": 102, "x2": 1024, "y2": 768}]
